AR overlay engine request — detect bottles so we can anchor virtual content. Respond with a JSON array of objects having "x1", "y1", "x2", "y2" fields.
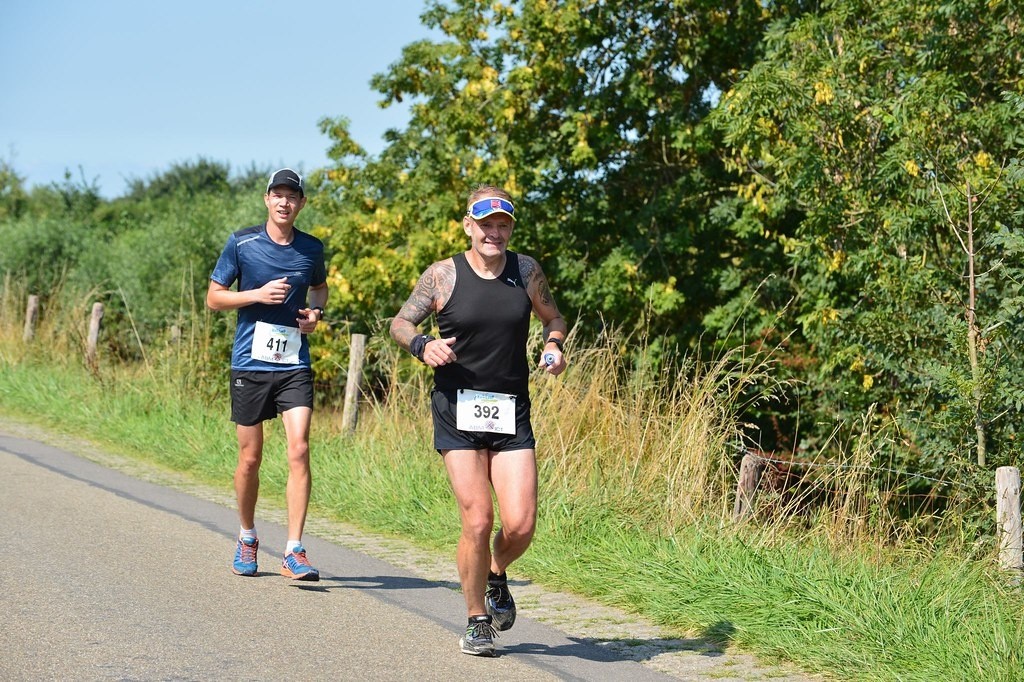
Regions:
[{"x1": 544, "y1": 354, "x2": 555, "y2": 368}]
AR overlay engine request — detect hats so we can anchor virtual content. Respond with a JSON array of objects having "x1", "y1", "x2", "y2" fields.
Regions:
[
  {"x1": 267, "y1": 168, "x2": 306, "y2": 198},
  {"x1": 468, "y1": 196, "x2": 517, "y2": 222}
]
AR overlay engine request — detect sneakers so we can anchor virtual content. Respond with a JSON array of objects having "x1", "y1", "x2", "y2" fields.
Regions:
[
  {"x1": 279, "y1": 546, "x2": 320, "y2": 582},
  {"x1": 460, "y1": 615, "x2": 497, "y2": 657},
  {"x1": 484, "y1": 575, "x2": 517, "y2": 631},
  {"x1": 230, "y1": 531, "x2": 259, "y2": 576}
]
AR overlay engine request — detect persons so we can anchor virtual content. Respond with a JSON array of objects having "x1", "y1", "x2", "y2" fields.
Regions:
[
  {"x1": 389, "y1": 185, "x2": 569, "y2": 657},
  {"x1": 207, "y1": 168, "x2": 329, "y2": 581}
]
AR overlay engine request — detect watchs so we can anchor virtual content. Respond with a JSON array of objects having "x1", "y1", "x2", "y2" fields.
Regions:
[
  {"x1": 311, "y1": 305, "x2": 324, "y2": 320},
  {"x1": 548, "y1": 338, "x2": 563, "y2": 352}
]
